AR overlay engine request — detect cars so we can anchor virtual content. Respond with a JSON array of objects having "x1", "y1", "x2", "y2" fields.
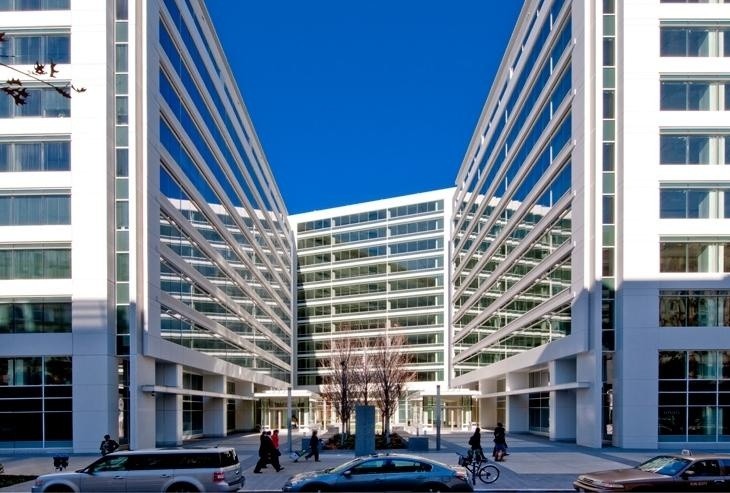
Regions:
[
  {"x1": 573, "y1": 452, "x2": 730, "y2": 493},
  {"x1": 282, "y1": 453, "x2": 474, "y2": 493}
]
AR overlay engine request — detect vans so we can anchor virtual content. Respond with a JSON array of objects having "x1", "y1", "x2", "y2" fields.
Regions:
[{"x1": 30, "y1": 445, "x2": 246, "y2": 493}]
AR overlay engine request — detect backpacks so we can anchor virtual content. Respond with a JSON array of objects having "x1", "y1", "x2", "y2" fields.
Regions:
[{"x1": 469, "y1": 433, "x2": 478, "y2": 445}]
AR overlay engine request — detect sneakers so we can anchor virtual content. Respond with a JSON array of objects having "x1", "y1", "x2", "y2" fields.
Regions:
[
  {"x1": 254, "y1": 465, "x2": 284, "y2": 474},
  {"x1": 476, "y1": 454, "x2": 509, "y2": 462},
  {"x1": 305, "y1": 456, "x2": 320, "y2": 462}
]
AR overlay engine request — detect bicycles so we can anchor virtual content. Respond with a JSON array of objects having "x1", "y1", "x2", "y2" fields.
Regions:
[{"x1": 456, "y1": 449, "x2": 501, "y2": 484}]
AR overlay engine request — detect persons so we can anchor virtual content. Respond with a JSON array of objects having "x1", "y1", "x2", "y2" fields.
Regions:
[
  {"x1": 468, "y1": 425, "x2": 486, "y2": 461},
  {"x1": 307, "y1": 432, "x2": 324, "y2": 461},
  {"x1": 493, "y1": 422, "x2": 505, "y2": 458},
  {"x1": 252, "y1": 428, "x2": 284, "y2": 473},
  {"x1": 99, "y1": 435, "x2": 121, "y2": 465},
  {"x1": 495, "y1": 426, "x2": 510, "y2": 455}
]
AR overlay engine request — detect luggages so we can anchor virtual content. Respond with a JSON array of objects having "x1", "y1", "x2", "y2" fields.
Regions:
[{"x1": 288, "y1": 450, "x2": 300, "y2": 463}]
